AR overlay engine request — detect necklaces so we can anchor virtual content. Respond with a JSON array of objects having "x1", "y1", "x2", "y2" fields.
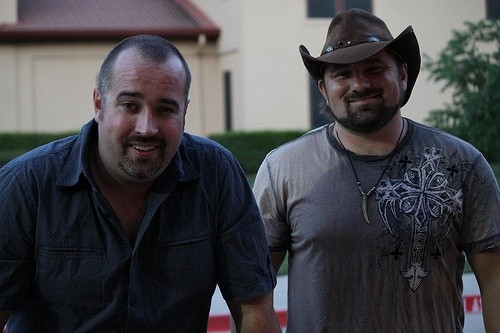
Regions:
[{"x1": 335, "y1": 116, "x2": 404, "y2": 225}]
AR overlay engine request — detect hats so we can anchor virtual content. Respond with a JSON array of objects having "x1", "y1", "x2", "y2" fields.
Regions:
[{"x1": 299, "y1": 8, "x2": 421, "y2": 109}]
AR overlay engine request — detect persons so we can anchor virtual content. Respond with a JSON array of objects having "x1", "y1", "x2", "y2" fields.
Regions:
[
  {"x1": 0, "y1": 34, "x2": 277, "y2": 333},
  {"x1": 231, "y1": 9, "x2": 500, "y2": 333}
]
